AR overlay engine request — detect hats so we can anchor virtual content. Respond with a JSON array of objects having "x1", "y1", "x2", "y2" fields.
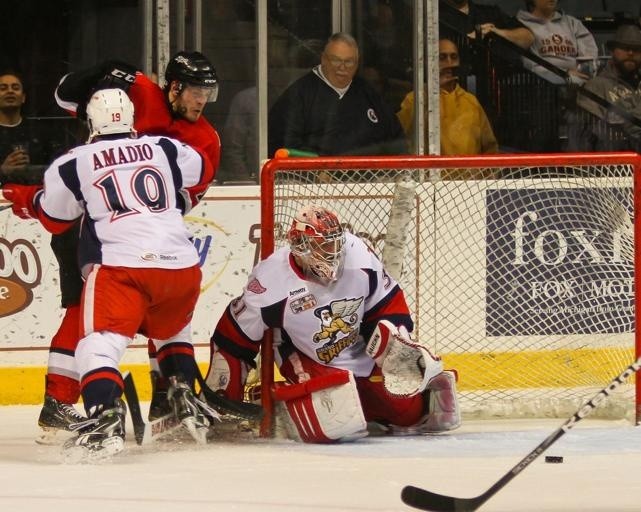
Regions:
[{"x1": 606, "y1": 23, "x2": 641, "y2": 52}]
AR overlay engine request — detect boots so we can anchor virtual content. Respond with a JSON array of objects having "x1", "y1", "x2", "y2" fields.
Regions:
[
  {"x1": 38, "y1": 392, "x2": 89, "y2": 431},
  {"x1": 149, "y1": 370, "x2": 214, "y2": 428},
  {"x1": 63, "y1": 397, "x2": 126, "y2": 450}
]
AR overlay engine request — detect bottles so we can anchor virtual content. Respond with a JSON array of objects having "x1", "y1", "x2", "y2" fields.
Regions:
[{"x1": 275, "y1": 148, "x2": 320, "y2": 162}]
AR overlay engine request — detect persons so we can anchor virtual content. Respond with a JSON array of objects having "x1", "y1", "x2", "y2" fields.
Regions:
[
  {"x1": 2, "y1": 89, "x2": 214, "y2": 452},
  {"x1": 0, "y1": 0, "x2": 641, "y2": 191},
  {"x1": 198, "y1": 205, "x2": 462, "y2": 445},
  {"x1": 37, "y1": 51, "x2": 220, "y2": 434}
]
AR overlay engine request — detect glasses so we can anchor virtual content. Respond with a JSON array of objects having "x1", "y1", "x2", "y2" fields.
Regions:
[{"x1": 324, "y1": 51, "x2": 355, "y2": 67}]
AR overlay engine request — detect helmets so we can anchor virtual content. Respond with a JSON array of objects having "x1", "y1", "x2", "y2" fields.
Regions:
[
  {"x1": 162, "y1": 51, "x2": 219, "y2": 103},
  {"x1": 85, "y1": 88, "x2": 138, "y2": 144},
  {"x1": 286, "y1": 205, "x2": 346, "y2": 282}
]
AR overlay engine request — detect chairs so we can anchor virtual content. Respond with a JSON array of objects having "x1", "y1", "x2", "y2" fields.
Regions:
[{"x1": 470, "y1": 1, "x2": 641, "y2": 81}]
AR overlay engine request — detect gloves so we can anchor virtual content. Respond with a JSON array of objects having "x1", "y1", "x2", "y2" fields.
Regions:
[
  {"x1": 77, "y1": 77, "x2": 129, "y2": 121},
  {"x1": 2, "y1": 184, "x2": 43, "y2": 219}
]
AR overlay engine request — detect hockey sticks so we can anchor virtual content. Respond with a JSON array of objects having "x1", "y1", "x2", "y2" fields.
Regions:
[
  {"x1": 187, "y1": 359, "x2": 264, "y2": 423},
  {"x1": 401, "y1": 357, "x2": 639, "y2": 512},
  {"x1": 123, "y1": 370, "x2": 187, "y2": 446}
]
